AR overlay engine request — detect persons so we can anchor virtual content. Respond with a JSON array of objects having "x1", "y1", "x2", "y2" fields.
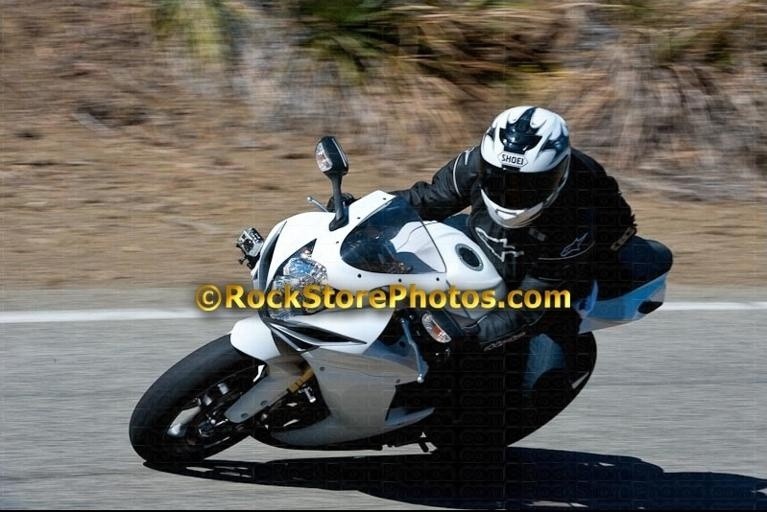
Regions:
[{"x1": 328, "y1": 105, "x2": 637, "y2": 480}]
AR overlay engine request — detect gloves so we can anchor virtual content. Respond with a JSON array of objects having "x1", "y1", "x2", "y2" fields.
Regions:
[
  {"x1": 418, "y1": 310, "x2": 481, "y2": 353},
  {"x1": 325, "y1": 192, "x2": 357, "y2": 210}
]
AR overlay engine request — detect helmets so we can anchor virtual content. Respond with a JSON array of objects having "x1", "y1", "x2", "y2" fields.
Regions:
[{"x1": 478, "y1": 106, "x2": 571, "y2": 229}]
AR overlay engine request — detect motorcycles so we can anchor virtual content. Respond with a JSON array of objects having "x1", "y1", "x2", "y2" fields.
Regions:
[{"x1": 128, "y1": 132, "x2": 675, "y2": 467}]
